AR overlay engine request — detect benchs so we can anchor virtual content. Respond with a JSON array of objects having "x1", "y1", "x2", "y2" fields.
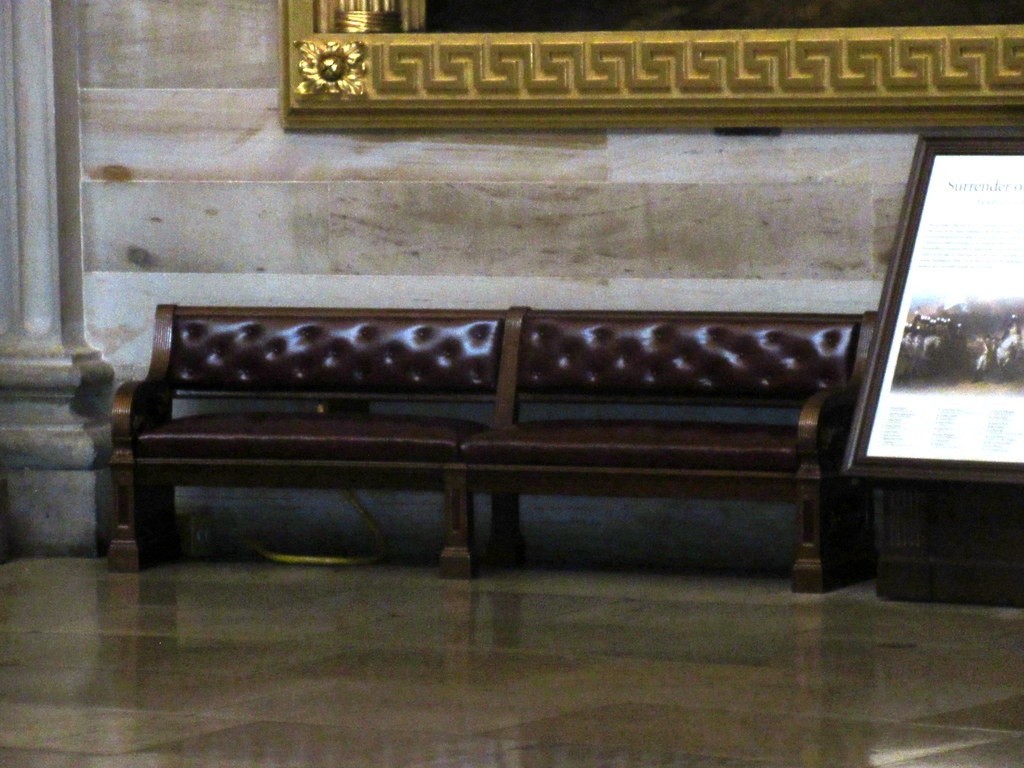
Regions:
[
  {"x1": 108, "y1": 303, "x2": 505, "y2": 579},
  {"x1": 461, "y1": 306, "x2": 877, "y2": 592}
]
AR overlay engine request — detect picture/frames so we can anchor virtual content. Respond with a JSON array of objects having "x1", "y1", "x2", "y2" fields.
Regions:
[
  {"x1": 278, "y1": 0, "x2": 1024, "y2": 136},
  {"x1": 841, "y1": 125, "x2": 1024, "y2": 485}
]
